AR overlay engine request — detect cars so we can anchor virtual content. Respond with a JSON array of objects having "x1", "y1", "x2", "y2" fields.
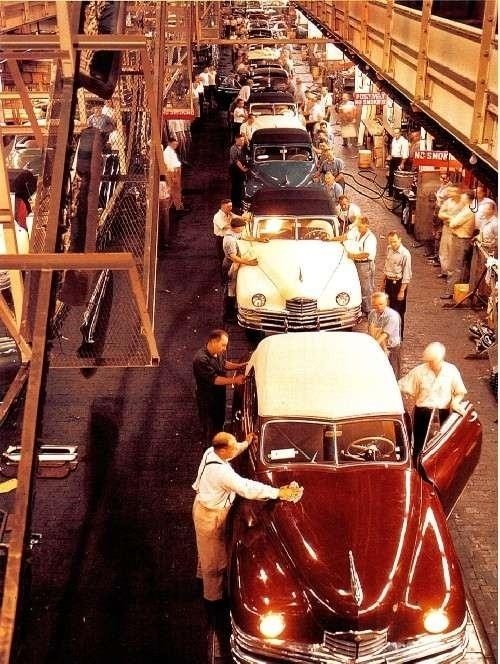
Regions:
[
  {"x1": 229, "y1": 331, "x2": 494, "y2": 663},
  {"x1": 244, "y1": 128, "x2": 326, "y2": 196},
  {"x1": 234, "y1": 187, "x2": 362, "y2": 334},
  {"x1": 239, "y1": 92, "x2": 309, "y2": 142},
  {"x1": 228, "y1": 1, "x2": 298, "y2": 95}
]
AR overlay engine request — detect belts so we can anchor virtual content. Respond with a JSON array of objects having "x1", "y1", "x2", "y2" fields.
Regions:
[{"x1": 354, "y1": 260, "x2": 374, "y2": 263}]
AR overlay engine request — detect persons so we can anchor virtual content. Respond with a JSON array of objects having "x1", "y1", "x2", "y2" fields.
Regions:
[
  {"x1": 368, "y1": 292, "x2": 402, "y2": 379},
  {"x1": 323, "y1": 216, "x2": 377, "y2": 315},
  {"x1": 86, "y1": 107, "x2": 114, "y2": 154},
  {"x1": 192, "y1": 329, "x2": 248, "y2": 446},
  {"x1": 397, "y1": 343, "x2": 468, "y2": 467},
  {"x1": 190, "y1": 15, "x2": 255, "y2": 207},
  {"x1": 430, "y1": 174, "x2": 498, "y2": 298},
  {"x1": 191, "y1": 432, "x2": 300, "y2": 620},
  {"x1": 335, "y1": 196, "x2": 362, "y2": 230},
  {"x1": 101, "y1": 99, "x2": 114, "y2": 120},
  {"x1": 381, "y1": 231, "x2": 412, "y2": 341},
  {"x1": 386, "y1": 128, "x2": 410, "y2": 197},
  {"x1": 222, "y1": 217, "x2": 270, "y2": 324},
  {"x1": 275, "y1": 37, "x2": 356, "y2": 208},
  {"x1": 213, "y1": 199, "x2": 249, "y2": 278},
  {"x1": 164, "y1": 136, "x2": 190, "y2": 213}
]
